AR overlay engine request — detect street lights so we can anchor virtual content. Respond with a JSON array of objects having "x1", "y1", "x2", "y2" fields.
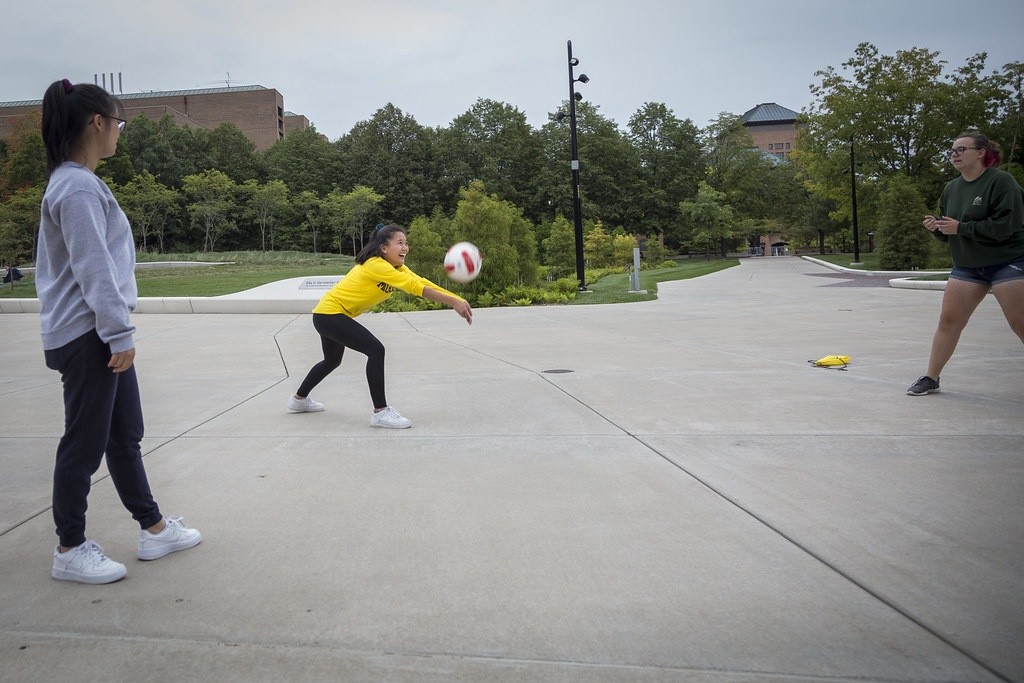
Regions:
[
  {"x1": 842, "y1": 133, "x2": 865, "y2": 270},
  {"x1": 554, "y1": 38, "x2": 590, "y2": 292}
]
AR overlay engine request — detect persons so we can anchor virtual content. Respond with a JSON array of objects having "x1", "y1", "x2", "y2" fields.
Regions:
[
  {"x1": 907, "y1": 133, "x2": 1024, "y2": 396},
  {"x1": 35, "y1": 78, "x2": 201, "y2": 584},
  {"x1": 287, "y1": 224, "x2": 472, "y2": 428},
  {"x1": 760, "y1": 240, "x2": 765, "y2": 256}
]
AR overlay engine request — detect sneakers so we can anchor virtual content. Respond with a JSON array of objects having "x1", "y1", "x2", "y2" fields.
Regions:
[
  {"x1": 287, "y1": 394, "x2": 325, "y2": 411},
  {"x1": 51, "y1": 540, "x2": 128, "y2": 585},
  {"x1": 906, "y1": 376, "x2": 943, "y2": 395},
  {"x1": 138, "y1": 516, "x2": 202, "y2": 560},
  {"x1": 370, "y1": 406, "x2": 413, "y2": 429}
]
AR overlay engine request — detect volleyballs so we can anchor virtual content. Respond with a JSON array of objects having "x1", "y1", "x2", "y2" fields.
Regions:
[{"x1": 443, "y1": 242, "x2": 483, "y2": 284}]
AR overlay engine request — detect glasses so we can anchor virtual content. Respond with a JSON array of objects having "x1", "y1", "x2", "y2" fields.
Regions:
[
  {"x1": 88, "y1": 113, "x2": 128, "y2": 135},
  {"x1": 945, "y1": 146, "x2": 981, "y2": 156}
]
